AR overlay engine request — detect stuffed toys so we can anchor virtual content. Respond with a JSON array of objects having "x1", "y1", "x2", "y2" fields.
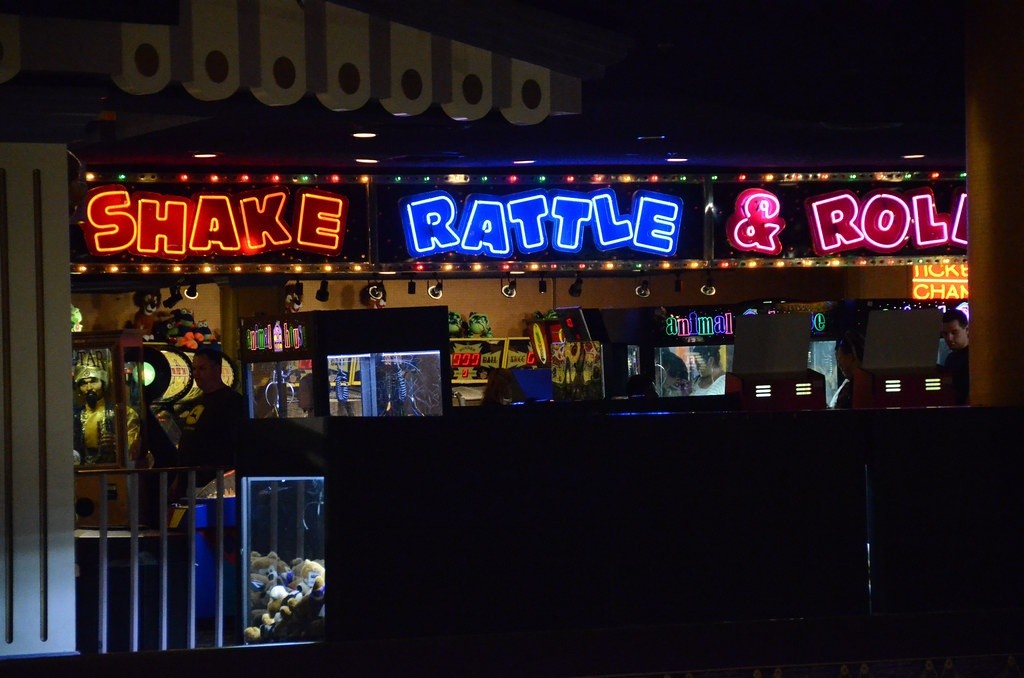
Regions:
[
  {"x1": 133, "y1": 292, "x2": 218, "y2": 349},
  {"x1": 243, "y1": 550, "x2": 325, "y2": 646}
]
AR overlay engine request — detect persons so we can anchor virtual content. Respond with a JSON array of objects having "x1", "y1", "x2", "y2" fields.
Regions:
[
  {"x1": 942, "y1": 308, "x2": 969, "y2": 367},
  {"x1": 829, "y1": 333, "x2": 865, "y2": 408},
  {"x1": 73, "y1": 350, "x2": 141, "y2": 463},
  {"x1": 654, "y1": 346, "x2": 726, "y2": 397},
  {"x1": 296, "y1": 373, "x2": 314, "y2": 417},
  {"x1": 167, "y1": 344, "x2": 246, "y2": 500}
]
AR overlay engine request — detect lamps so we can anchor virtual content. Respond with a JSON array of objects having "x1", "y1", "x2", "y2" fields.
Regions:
[
  {"x1": 569, "y1": 277, "x2": 583, "y2": 297},
  {"x1": 162, "y1": 285, "x2": 183, "y2": 309},
  {"x1": 501, "y1": 279, "x2": 518, "y2": 297},
  {"x1": 185, "y1": 282, "x2": 199, "y2": 299},
  {"x1": 674, "y1": 277, "x2": 681, "y2": 292},
  {"x1": 370, "y1": 283, "x2": 383, "y2": 299},
  {"x1": 427, "y1": 278, "x2": 443, "y2": 300},
  {"x1": 143, "y1": 291, "x2": 157, "y2": 315},
  {"x1": 636, "y1": 278, "x2": 650, "y2": 297},
  {"x1": 701, "y1": 277, "x2": 716, "y2": 295},
  {"x1": 538, "y1": 278, "x2": 546, "y2": 293},
  {"x1": 407, "y1": 280, "x2": 416, "y2": 295},
  {"x1": 314, "y1": 280, "x2": 330, "y2": 301}
]
